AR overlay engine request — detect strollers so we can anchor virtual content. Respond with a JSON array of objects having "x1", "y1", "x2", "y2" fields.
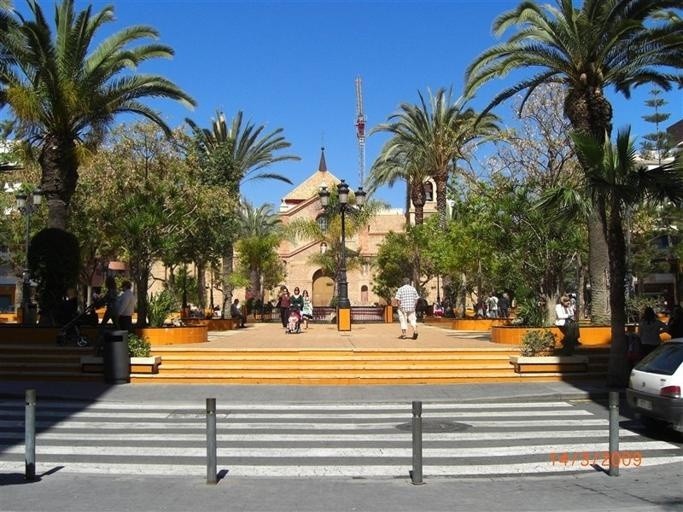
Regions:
[
  {"x1": 285, "y1": 304, "x2": 301, "y2": 335},
  {"x1": 54, "y1": 300, "x2": 102, "y2": 348}
]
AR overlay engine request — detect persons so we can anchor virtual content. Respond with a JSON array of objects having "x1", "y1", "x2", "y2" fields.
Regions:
[
  {"x1": 97, "y1": 275, "x2": 120, "y2": 330},
  {"x1": 415, "y1": 291, "x2": 522, "y2": 326},
  {"x1": 666, "y1": 304, "x2": 683, "y2": 339},
  {"x1": 226, "y1": 285, "x2": 313, "y2": 334},
  {"x1": 637, "y1": 306, "x2": 671, "y2": 360},
  {"x1": 108, "y1": 279, "x2": 137, "y2": 336},
  {"x1": 552, "y1": 295, "x2": 583, "y2": 347},
  {"x1": 392, "y1": 276, "x2": 420, "y2": 340}
]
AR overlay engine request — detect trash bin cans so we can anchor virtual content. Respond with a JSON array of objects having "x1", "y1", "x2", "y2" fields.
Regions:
[{"x1": 103, "y1": 330, "x2": 129, "y2": 384}]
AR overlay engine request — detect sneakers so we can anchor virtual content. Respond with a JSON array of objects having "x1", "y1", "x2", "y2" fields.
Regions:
[
  {"x1": 399, "y1": 335, "x2": 406, "y2": 339},
  {"x1": 412, "y1": 332, "x2": 417, "y2": 339}
]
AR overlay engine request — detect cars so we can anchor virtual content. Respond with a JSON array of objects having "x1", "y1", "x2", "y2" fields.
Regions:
[
  {"x1": 429, "y1": 300, "x2": 486, "y2": 319},
  {"x1": 624, "y1": 337, "x2": 682, "y2": 441},
  {"x1": 165, "y1": 301, "x2": 243, "y2": 319}
]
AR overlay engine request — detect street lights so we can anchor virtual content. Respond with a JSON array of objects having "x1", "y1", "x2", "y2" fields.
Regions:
[
  {"x1": 316, "y1": 179, "x2": 366, "y2": 334},
  {"x1": 11, "y1": 180, "x2": 46, "y2": 328}
]
